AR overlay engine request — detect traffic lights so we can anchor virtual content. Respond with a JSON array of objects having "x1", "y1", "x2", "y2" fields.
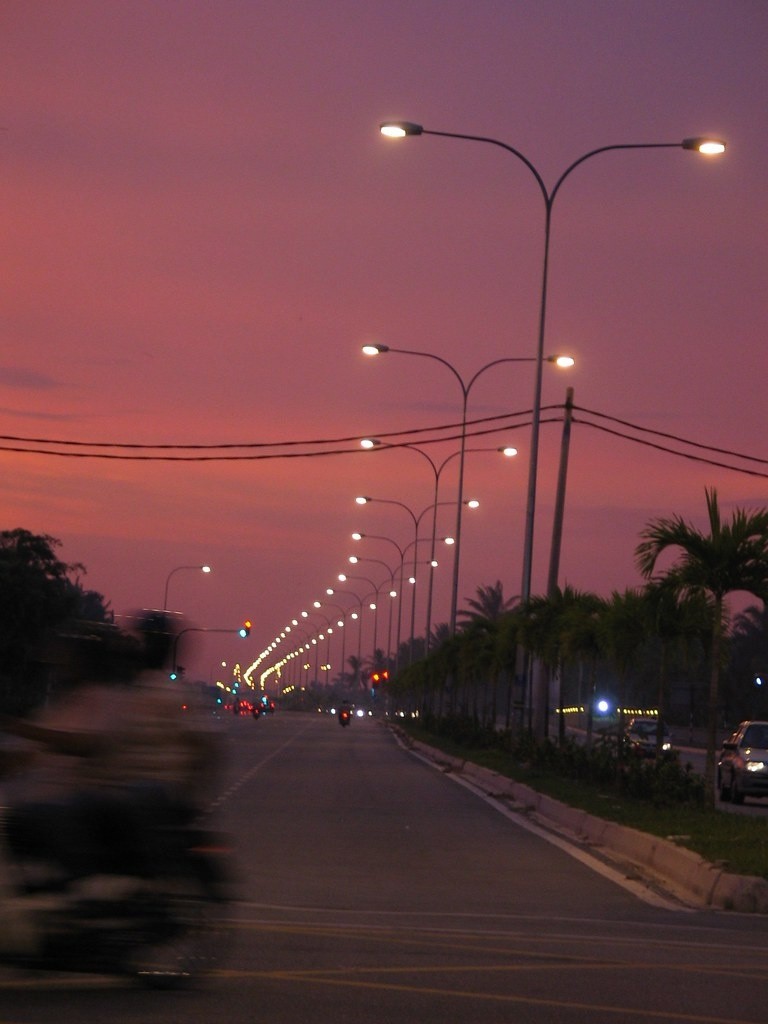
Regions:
[{"x1": 177, "y1": 667, "x2": 185, "y2": 678}]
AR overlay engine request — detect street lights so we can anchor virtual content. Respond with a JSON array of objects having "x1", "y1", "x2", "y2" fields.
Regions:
[
  {"x1": 242, "y1": 532, "x2": 456, "y2": 699},
  {"x1": 354, "y1": 495, "x2": 482, "y2": 667},
  {"x1": 163, "y1": 564, "x2": 212, "y2": 611},
  {"x1": 363, "y1": 341, "x2": 578, "y2": 640},
  {"x1": 380, "y1": 119, "x2": 730, "y2": 629},
  {"x1": 358, "y1": 435, "x2": 519, "y2": 659}
]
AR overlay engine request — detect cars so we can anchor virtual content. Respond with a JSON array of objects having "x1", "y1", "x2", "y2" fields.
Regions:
[
  {"x1": 716, "y1": 720, "x2": 768, "y2": 804},
  {"x1": 622, "y1": 717, "x2": 672, "y2": 756}
]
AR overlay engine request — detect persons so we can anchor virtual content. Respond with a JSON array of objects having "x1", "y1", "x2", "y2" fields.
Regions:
[
  {"x1": 233, "y1": 697, "x2": 354, "y2": 726},
  {"x1": 0, "y1": 610, "x2": 242, "y2": 980}
]
